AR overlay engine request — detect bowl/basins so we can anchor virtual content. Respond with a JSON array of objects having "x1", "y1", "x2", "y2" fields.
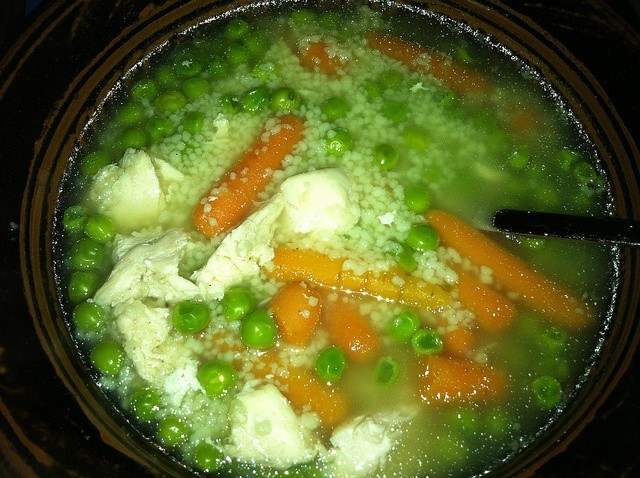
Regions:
[{"x1": 18, "y1": 0, "x2": 636, "y2": 477}]
